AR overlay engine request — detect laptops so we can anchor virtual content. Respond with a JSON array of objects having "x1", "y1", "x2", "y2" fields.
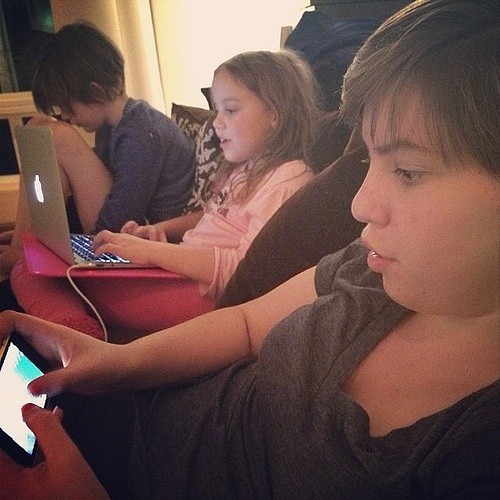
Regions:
[{"x1": 15, "y1": 124, "x2": 158, "y2": 268}]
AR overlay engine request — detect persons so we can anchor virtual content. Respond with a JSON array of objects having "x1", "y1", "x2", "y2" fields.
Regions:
[
  {"x1": 10, "y1": 51, "x2": 315, "y2": 342},
  {"x1": 0, "y1": 23, "x2": 196, "y2": 274},
  {"x1": 0, "y1": 0, "x2": 500, "y2": 500}
]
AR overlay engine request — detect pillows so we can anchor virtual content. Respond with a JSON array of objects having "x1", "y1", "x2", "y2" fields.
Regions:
[{"x1": 169, "y1": 102, "x2": 225, "y2": 216}]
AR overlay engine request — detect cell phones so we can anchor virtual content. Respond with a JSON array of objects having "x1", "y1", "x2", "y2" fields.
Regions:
[{"x1": 0, "y1": 329, "x2": 56, "y2": 469}]
